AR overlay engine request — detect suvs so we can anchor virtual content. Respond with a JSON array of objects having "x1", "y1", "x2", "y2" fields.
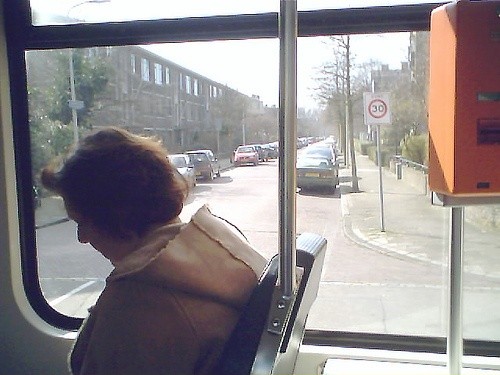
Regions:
[{"x1": 187, "y1": 148, "x2": 220, "y2": 181}]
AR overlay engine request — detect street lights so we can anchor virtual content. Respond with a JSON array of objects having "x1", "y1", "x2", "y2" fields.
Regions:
[{"x1": 65, "y1": 0, "x2": 112, "y2": 141}]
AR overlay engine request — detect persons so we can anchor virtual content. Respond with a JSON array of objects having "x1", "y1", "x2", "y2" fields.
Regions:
[{"x1": 35, "y1": 126, "x2": 273, "y2": 375}]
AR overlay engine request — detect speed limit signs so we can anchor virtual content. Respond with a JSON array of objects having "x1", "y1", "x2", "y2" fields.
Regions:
[{"x1": 364, "y1": 92, "x2": 391, "y2": 124}]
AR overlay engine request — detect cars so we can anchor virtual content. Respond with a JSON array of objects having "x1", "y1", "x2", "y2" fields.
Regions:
[
  {"x1": 233, "y1": 135, "x2": 339, "y2": 193},
  {"x1": 168, "y1": 154, "x2": 196, "y2": 189}
]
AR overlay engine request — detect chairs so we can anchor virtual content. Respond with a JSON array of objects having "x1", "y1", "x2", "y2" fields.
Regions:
[{"x1": 214, "y1": 230, "x2": 328, "y2": 375}]
